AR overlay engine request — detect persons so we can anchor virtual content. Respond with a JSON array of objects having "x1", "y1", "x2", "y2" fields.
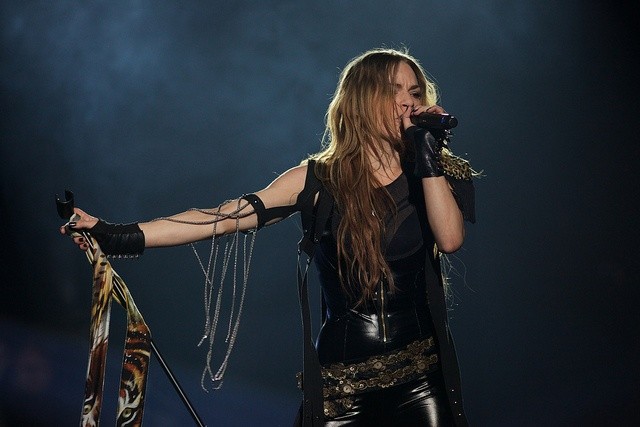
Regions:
[{"x1": 60, "y1": 46, "x2": 484, "y2": 427}]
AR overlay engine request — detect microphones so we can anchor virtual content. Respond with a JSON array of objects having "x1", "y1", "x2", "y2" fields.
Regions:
[{"x1": 409, "y1": 112, "x2": 458, "y2": 129}]
